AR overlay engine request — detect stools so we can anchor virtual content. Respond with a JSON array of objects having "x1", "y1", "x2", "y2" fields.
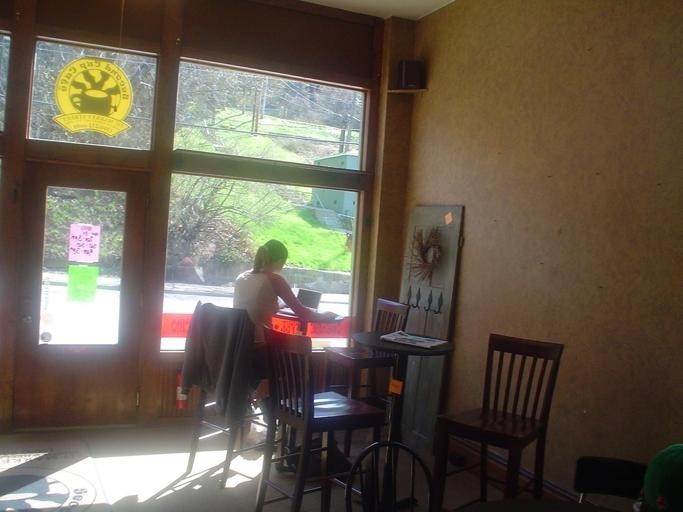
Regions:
[{"x1": 325, "y1": 299, "x2": 412, "y2": 477}]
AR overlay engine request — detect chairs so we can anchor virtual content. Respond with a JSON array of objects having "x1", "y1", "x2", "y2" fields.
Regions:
[
  {"x1": 344, "y1": 439, "x2": 683, "y2": 512},
  {"x1": 184, "y1": 299, "x2": 285, "y2": 489},
  {"x1": 431, "y1": 333, "x2": 564, "y2": 509},
  {"x1": 255, "y1": 326, "x2": 386, "y2": 510}
]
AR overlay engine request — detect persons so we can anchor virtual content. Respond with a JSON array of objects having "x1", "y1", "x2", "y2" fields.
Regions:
[{"x1": 226, "y1": 238, "x2": 338, "y2": 430}]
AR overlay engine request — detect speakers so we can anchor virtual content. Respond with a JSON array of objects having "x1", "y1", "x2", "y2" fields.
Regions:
[{"x1": 396, "y1": 58, "x2": 424, "y2": 89}]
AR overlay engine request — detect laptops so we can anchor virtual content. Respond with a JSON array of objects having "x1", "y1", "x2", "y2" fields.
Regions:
[{"x1": 279, "y1": 288, "x2": 323, "y2": 315}]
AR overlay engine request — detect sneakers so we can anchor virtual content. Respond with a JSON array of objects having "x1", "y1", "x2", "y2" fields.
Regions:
[{"x1": 259, "y1": 398, "x2": 271, "y2": 424}]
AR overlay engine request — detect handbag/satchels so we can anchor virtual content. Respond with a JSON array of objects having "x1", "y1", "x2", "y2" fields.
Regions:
[{"x1": 285, "y1": 436, "x2": 352, "y2": 479}]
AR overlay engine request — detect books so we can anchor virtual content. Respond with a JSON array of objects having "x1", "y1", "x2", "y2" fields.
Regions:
[{"x1": 378, "y1": 329, "x2": 450, "y2": 350}]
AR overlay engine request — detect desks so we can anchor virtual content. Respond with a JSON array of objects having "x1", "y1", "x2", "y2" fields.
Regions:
[
  {"x1": 349, "y1": 329, "x2": 455, "y2": 500},
  {"x1": 271, "y1": 302, "x2": 345, "y2": 338}
]
